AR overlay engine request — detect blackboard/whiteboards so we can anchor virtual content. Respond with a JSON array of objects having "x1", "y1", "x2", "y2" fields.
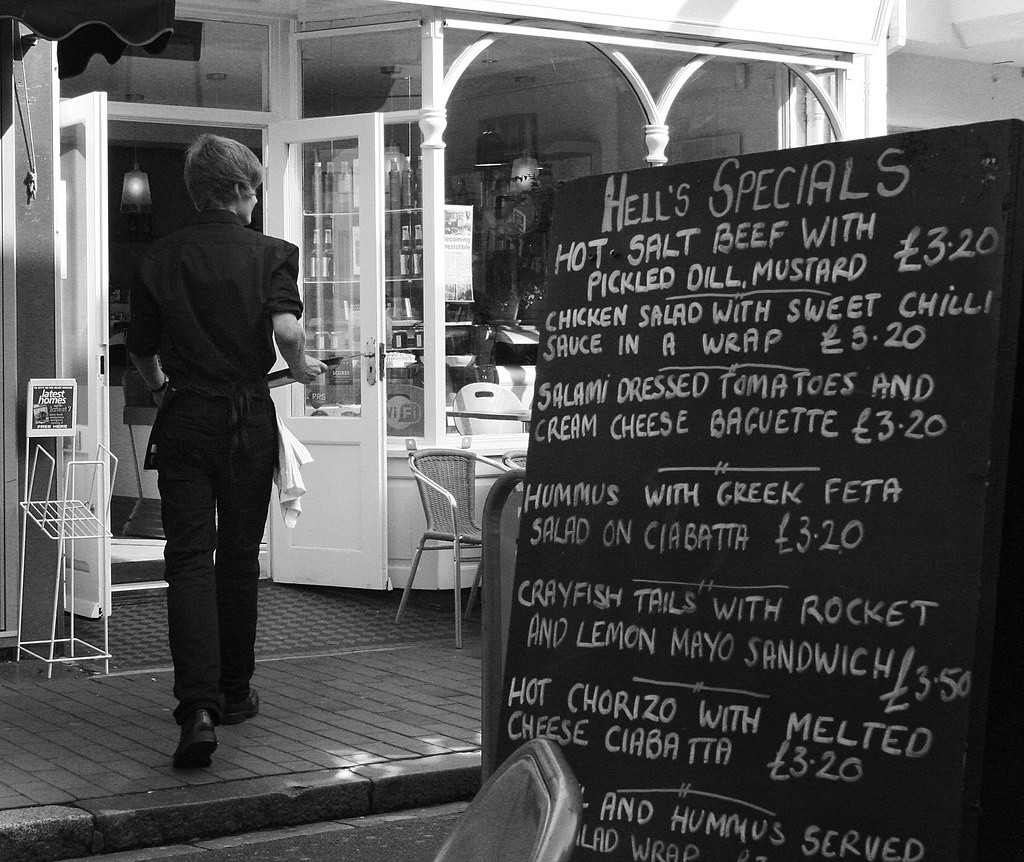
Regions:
[{"x1": 489, "y1": 121, "x2": 1023, "y2": 862}]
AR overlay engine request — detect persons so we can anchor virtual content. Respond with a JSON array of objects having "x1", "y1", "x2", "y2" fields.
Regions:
[{"x1": 128, "y1": 132, "x2": 332, "y2": 772}]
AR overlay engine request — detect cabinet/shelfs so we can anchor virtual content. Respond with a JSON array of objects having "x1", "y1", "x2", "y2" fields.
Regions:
[{"x1": 301, "y1": 205, "x2": 424, "y2": 399}]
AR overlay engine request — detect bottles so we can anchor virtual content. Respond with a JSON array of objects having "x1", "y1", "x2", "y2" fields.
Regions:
[{"x1": 288, "y1": 152, "x2": 553, "y2": 416}]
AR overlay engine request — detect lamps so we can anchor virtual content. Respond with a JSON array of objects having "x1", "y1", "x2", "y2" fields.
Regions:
[
  {"x1": 118, "y1": 124, "x2": 154, "y2": 215},
  {"x1": 508, "y1": 155, "x2": 540, "y2": 196}
]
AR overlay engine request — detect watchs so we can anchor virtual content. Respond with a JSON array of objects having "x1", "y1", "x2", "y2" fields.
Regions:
[{"x1": 149, "y1": 373, "x2": 170, "y2": 396}]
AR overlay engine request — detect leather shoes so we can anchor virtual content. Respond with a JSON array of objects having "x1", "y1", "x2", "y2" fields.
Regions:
[
  {"x1": 173, "y1": 711, "x2": 218, "y2": 768},
  {"x1": 218, "y1": 687, "x2": 260, "y2": 724}
]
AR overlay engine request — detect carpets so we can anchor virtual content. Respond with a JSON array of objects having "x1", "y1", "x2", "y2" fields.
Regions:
[{"x1": 111, "y1": 559, "x2": 167, "y2": 585}]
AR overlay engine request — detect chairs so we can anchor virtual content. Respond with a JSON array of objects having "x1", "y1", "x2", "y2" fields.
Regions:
[{"x1": 394, "y1": 383, "x2": 528, "y2": 648}]
